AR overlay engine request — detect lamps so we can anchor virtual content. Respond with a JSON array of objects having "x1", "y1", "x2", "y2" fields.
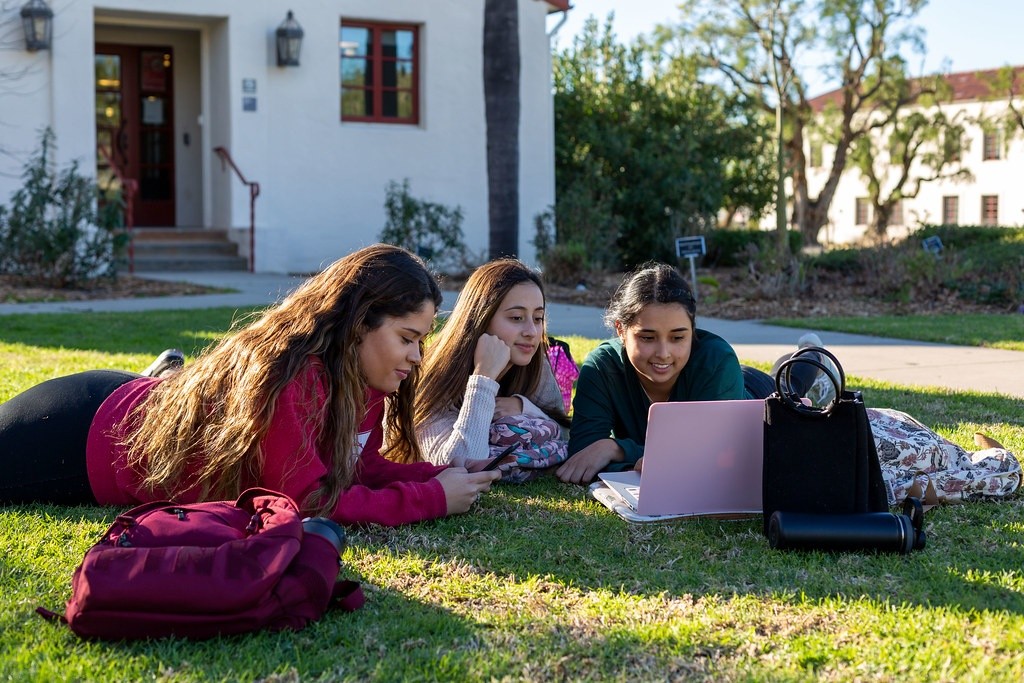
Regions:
[
  {"x1": 20, "y1": 0, "x2": 55, "y2": 52},
  {"x1": 277, "y1": 11, "x2": 304, "y2": 66}
]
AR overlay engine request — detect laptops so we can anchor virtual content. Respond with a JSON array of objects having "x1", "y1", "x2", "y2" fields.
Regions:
[{"x1": 598, "y1": 399, "x2": 763, "y2": 516}]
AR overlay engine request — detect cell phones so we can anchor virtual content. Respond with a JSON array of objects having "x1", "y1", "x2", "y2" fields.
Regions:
[{"x1": 480, "y1": 442, "x2": 521, "y2": 472}]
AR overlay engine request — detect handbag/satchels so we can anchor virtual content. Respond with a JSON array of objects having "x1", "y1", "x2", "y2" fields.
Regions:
[
  {"x1": 761, "y1": 348, "x2": 890, "y2": 514},
  {"x1": 489, "y1": 414, "x2": 569, "y2": 481},
  {"x1": 36, "y1": 487, "x2": 365, "y2": 643},
  {"x1": 545, "y1": 337, "x2": 579, "y2": 413}
]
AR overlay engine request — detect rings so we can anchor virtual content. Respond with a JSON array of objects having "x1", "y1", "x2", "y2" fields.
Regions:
[{"x1": 476, "y1": 494, "x2": 480, "y2": 502}]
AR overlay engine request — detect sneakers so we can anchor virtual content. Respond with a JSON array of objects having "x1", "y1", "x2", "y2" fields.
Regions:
[
  {"x1": 140, "y1": 348, "x2": 184, "y2": 378},
  {"x1": 799, "y1": 333, "x2": 841, "y2": 405}
]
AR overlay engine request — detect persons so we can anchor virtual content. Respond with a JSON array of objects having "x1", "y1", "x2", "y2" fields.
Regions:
[
  {"x1": 0, "y1": 244, "x2": 518, "y2": 526},
  {"x1": 556, "y1": 266, "x2": 842, "y2": 484},
  {"x1": 140, "y1": 257, "x2": 565, "y2": 466}
]
